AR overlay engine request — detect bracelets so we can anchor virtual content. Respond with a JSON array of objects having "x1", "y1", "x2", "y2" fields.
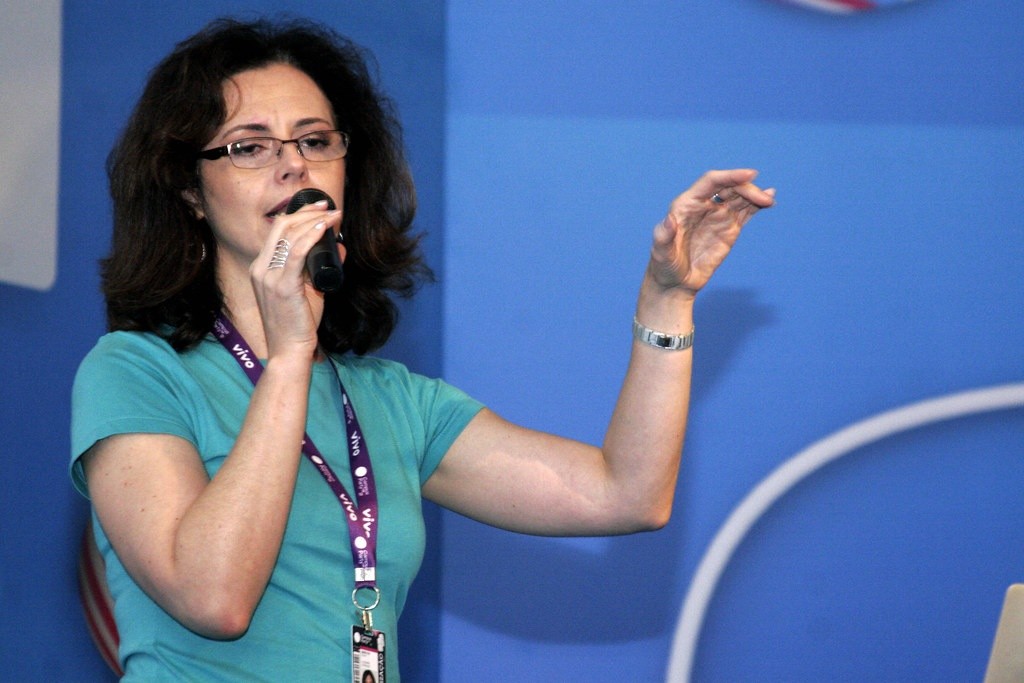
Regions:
[{"x1": 632, "y1": 316, "x2": 695, "y2": 351}]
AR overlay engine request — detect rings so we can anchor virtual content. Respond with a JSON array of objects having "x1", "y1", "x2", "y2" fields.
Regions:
[
  {"x1": 266, "y1": 238, "x2": 291, "y2": 270},
  {"x1": 712, "y1": 193, "x2": 725, "y2": 203}
]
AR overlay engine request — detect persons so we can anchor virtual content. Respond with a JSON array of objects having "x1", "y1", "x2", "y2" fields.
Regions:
[{"x1": 66, "y1": 17, "x2": 776, "y2": 683}]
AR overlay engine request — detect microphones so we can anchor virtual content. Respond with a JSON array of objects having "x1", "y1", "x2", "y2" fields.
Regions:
[{"x1": 287, "y1": 188, "x2": 345, "y2": 292}]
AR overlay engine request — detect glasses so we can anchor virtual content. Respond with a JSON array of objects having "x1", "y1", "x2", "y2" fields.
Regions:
[{"x1": 195, "y1": 127, "x2": 352, "y2": 169}]
